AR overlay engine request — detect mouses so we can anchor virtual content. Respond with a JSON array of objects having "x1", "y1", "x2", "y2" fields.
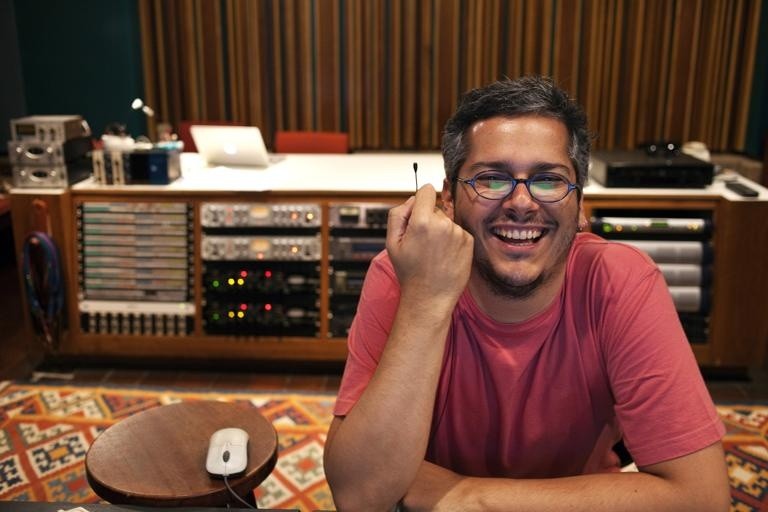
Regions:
[{"x1": 206, "y1": 427, "x2": 251, "y2": 478}]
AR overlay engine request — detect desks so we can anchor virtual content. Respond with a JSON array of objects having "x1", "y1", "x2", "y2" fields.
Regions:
[{"x1": 84, "y1": 400, "x2": 278, "y2": 505}]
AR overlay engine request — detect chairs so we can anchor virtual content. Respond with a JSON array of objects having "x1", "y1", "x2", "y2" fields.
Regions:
[{"x1": 273, "y1": 131, "x2": 351, "y2": 152}]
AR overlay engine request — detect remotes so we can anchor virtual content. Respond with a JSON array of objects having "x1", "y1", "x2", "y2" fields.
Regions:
[{"x1": 727, "y1": 182, "x2": 759, "y2": 197}]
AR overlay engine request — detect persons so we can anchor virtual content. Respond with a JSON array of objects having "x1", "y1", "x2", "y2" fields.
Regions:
[{"x1": 322, "y1": 74, "x2": 734, "y2": 512}]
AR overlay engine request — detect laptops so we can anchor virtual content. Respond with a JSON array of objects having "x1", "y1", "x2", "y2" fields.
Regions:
[{"x1": 191, "y1": 125, "x2": 285, "y2": 167}]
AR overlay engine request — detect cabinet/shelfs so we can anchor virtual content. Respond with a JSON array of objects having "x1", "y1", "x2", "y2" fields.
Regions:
[{"x1": 1, "y1": 151, "x2": 767, "y2": 369}]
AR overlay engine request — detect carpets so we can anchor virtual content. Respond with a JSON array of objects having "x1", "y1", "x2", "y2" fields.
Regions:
[{"x1": 0, "y1": 379, "x2": 768, "y2": 510}]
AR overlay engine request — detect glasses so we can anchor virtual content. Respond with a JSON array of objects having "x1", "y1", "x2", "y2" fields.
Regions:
[{"x1": 452, "y1": 170, "x2": 578, "y2": 203}]
